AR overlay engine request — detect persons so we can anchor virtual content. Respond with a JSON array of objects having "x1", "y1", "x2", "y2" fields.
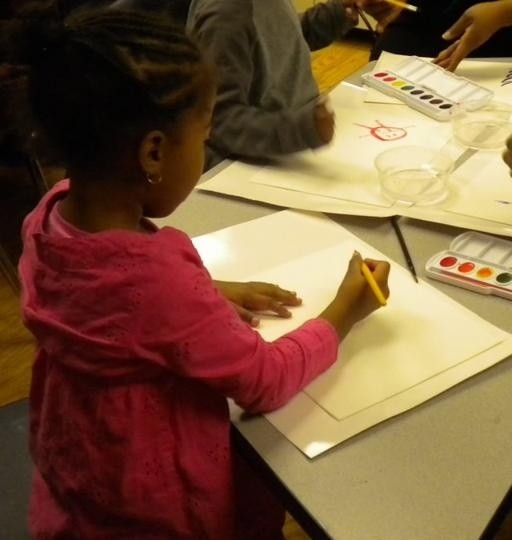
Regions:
[
  {"x1": 355, "y1": 0, "x2": 512, "y2": 72},
  {"x1": 185, "y1": 0, "x2": 358, "y2": 175},
  {"x1": 18, "y1": 9, "x2": 390, "y2": 540}
]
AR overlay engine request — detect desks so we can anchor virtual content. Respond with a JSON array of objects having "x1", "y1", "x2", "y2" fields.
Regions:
[{"x1": 141, "y1": 60, "x2": 512, "y2": 540}]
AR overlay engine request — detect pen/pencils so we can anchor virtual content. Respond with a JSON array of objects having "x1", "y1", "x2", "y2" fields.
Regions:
[
  {"x1": 354, "y1": 250, "x2": 387, "y2": 307},
  {"x1": 353, "y1": 3, "x2": 377, "y2": 41},
  {"x1": 390, "y1": 216, "x2": 419, "y2": 283},
  {"x1": 387, "y1": 0, "x2": 422, "y2": 13}
]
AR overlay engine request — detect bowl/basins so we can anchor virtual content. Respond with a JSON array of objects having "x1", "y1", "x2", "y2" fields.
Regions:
[
  {"x1": 449, "y1": 99, "x2": 512, "y2": 150},
  {"x1": 373, "y1": 144, "x2": 455, "y2": 206}
]
